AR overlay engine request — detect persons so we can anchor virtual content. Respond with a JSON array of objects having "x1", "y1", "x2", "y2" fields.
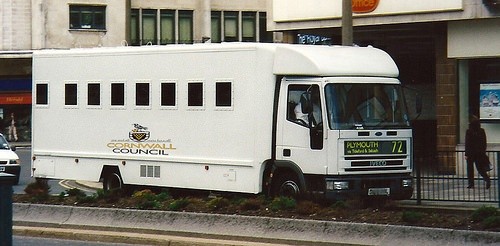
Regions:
[
  {"x1": 465, "y1": 114, "x2": 492, "y2": 189},
  {"x1": 294, "y1": 92, "x2": 321, "y2": 124},
  {"x1": 7, "y1": 112, "x2": 18, "y2": 142}
]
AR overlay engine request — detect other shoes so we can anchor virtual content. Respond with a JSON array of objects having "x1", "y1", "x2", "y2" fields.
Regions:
[
  {"x1": 466, "y1": 185, "x2": 474, "y2": 189},
  {"x1": 485, "y1": 179, "x2": 491, "y2": 189}
]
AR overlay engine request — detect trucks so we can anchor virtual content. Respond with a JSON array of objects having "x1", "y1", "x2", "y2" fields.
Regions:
[{"x1": 29, "y1": 41, "x2": 415, "y2": 208}]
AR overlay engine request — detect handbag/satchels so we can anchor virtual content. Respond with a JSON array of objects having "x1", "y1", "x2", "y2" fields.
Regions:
[{"x1": 477, "y1": 153, "x2": 490, "y2": 172}]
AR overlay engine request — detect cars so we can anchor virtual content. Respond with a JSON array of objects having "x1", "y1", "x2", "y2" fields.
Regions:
[{"x1": 0, "y1": 133, "x2": 21, "y2": 186}]
